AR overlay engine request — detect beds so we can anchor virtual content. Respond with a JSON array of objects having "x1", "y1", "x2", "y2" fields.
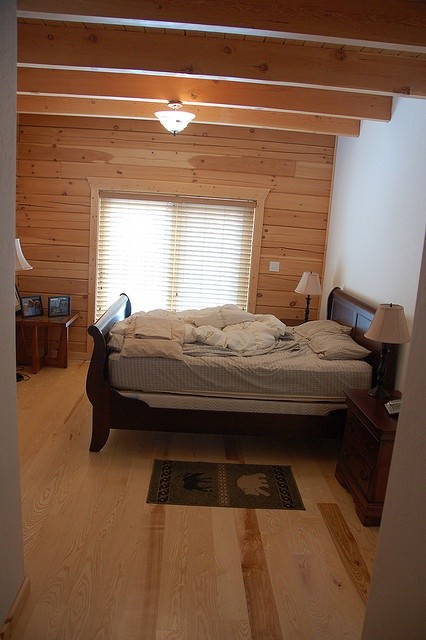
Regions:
[{"x1": 86, "y1": 287, "x2": 400, "y2": 452}]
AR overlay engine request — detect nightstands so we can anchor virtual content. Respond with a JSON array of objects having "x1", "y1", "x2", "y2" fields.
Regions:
[
  {"x1": 280, "y1": 319, "x2": 313, "y2": 326},
  {"x1": 335, "y1": 390, "x2": 402, "y2": 527}
]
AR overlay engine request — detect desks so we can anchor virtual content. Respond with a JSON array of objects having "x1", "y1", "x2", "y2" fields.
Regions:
[{"x1": 16, "y1": 312, "x2": 79, "y2": 374}]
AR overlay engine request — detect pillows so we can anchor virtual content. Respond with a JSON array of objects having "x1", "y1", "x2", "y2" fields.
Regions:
[
  {"x1": 294, "y1": 320, "x2": 353, "y2": 341},
  {"x1": 308, "y1": 334, "x2": 371, "y2": 360},
  {"x1": 121, "y1": 316, "x2": 185, "y2": 361}
]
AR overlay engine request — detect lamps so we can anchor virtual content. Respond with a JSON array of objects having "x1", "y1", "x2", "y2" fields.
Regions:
[
  {"x1": 364, "y1": 303, "x2": 412, "y2": 398},
  {"x1": 294, "y1": 271, "x2": 322, "y2": 322},
  {"x1": 15, "y1": 239, "x2": 33, "y2": 271},
  {"x1": 154, "y1": 101, "x2": 196, "y2": 136}
]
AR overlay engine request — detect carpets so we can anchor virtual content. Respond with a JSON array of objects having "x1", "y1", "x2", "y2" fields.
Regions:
[{"x1": 146, "y1": 459, "x2": 305, "y2": 510}]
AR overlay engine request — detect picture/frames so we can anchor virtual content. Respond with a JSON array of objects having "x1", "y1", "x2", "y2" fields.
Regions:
[
  {"x1": 48, "y1": 296, "x2": 71, "y2": 317},
  {"x1": 15, "y1": 283, "x2": 22, "y2": 315},
  {"x1": 20, "y1": 295, "x2": 44, "y2": 318}
]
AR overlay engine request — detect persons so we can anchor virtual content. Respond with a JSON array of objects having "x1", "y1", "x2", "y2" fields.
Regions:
[
  {"x1": 23, "y1": 303, "x2": 30, "y2": 316},
  {"x1": 33, "y1": 300, "x2": 41, "y2": 315},
  {"x1": 28, "y1": 300, "x2": 35, "y2": 315}
]
